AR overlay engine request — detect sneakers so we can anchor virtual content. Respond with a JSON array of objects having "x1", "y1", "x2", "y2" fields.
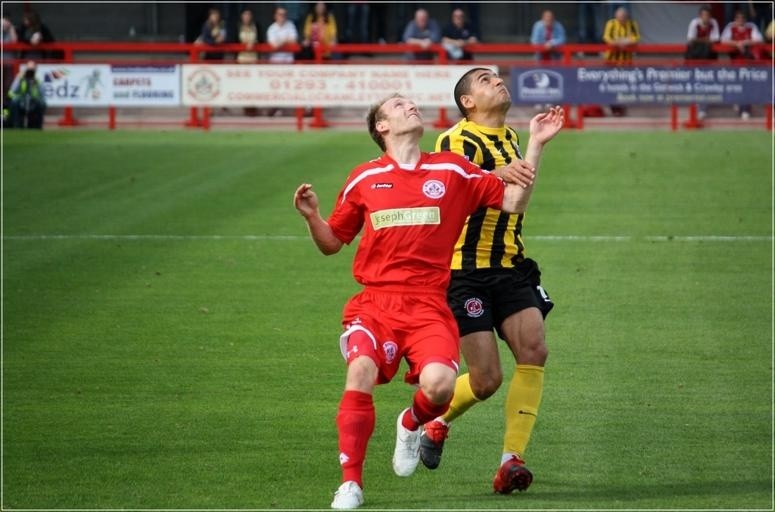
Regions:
[
  {"x1": 331, "y1": 481, "x2": 364, "y2": 509},
  {"x1": 393, "y1": 407, "x2": 421, "y2": 477},
  {"x1": 421, "y1": 417, "x2": 450, "y2": 469},
  {"x1": 494, "y1": 455, "x2": 532, "y2": 493}
]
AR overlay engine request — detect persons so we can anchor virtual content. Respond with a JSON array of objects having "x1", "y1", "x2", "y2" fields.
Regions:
[
  {"x1": 195, "y1": 4, "x2": 345, "y2": 125},
  {"x1": 602, "y1": 5, "x2": 642, "y2": 115},
  {"x1": 422, "y1": 68, "x2": 549, "y2": 493},
  {"x1": 7, "y1": 60, "x2": 46, "y2": 127},
  {"x1": 682, "y1": 4, "x2": 775, "y2": 123},
  {"x1": 398, "y1": 7, "x2": 588, "y2": 119},
  {"x1": 20, "y1": 12, "x2": 55, "y2": 45},
  {"x1": 0, "y1": 18, "x2": 20, "y2": 127},
  {"x1": 294, "y1": 96, "x2": 564, "y2": 508}
]
